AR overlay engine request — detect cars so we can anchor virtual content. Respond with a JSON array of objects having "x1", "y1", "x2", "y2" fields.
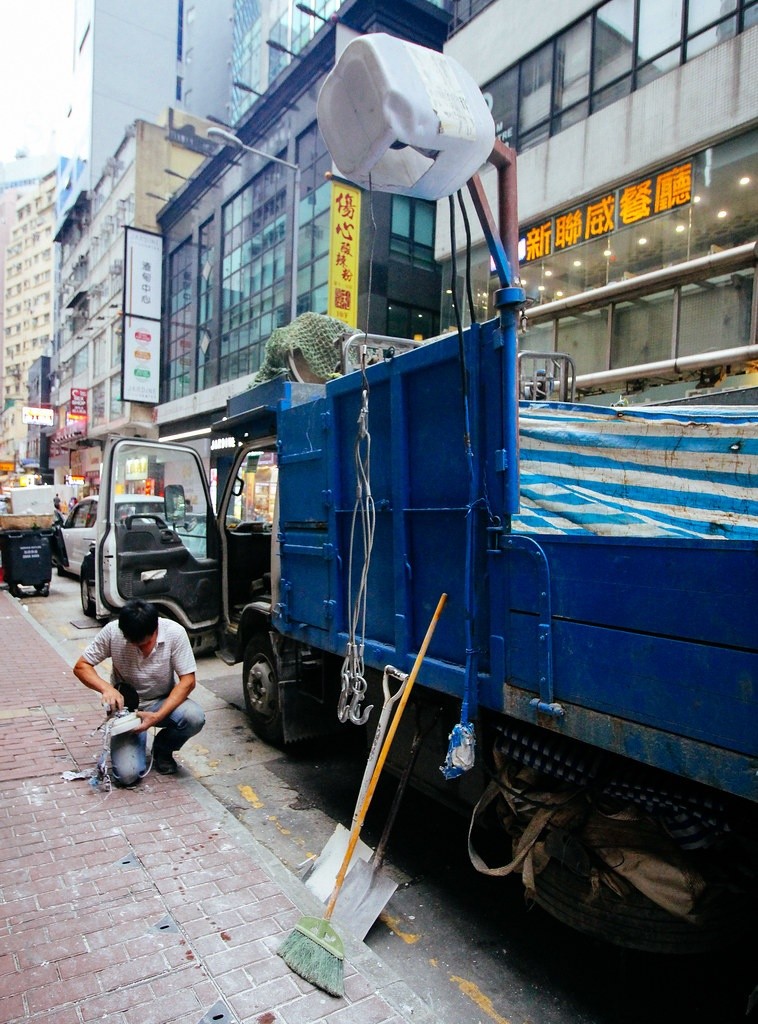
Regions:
[
  {"x1": 54, "y1": 494, "x2": 165, "y2": 580},
  {"x1": 0, "y1": 495, "x2": 66, "y2": 567},
  {"x1": 79, "y1": 512, "x2": 244, "y2": 617},
  {"x1": 0, "y1": 494, "x2": 12, "y2": 514}
]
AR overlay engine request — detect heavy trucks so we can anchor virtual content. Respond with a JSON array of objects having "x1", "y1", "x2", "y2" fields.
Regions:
[{"x1": 96, "y1": 33, "x2": 758, "y2": 961}]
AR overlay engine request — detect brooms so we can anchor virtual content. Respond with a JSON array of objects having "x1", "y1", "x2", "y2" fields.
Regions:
[{"x1": 275, "y1": 592, "x2": 449, "y2": 999}]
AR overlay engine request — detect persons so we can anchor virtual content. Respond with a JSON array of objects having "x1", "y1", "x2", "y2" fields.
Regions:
[
  {"x1": 54, "y1": 494, "x2": 60, "y2": 509},
  {"x1": 74, "y1": 599, "x2": 205, "y2": 785}
]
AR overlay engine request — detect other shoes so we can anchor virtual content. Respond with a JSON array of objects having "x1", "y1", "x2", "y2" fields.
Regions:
[{"x1": 151, "y1": 737, "x2": 178, "y2": 775}]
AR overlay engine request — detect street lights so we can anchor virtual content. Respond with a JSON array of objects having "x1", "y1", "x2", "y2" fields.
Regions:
[{"x1": 206, "y1": 127, "x2": 300, "y2": 326}]
